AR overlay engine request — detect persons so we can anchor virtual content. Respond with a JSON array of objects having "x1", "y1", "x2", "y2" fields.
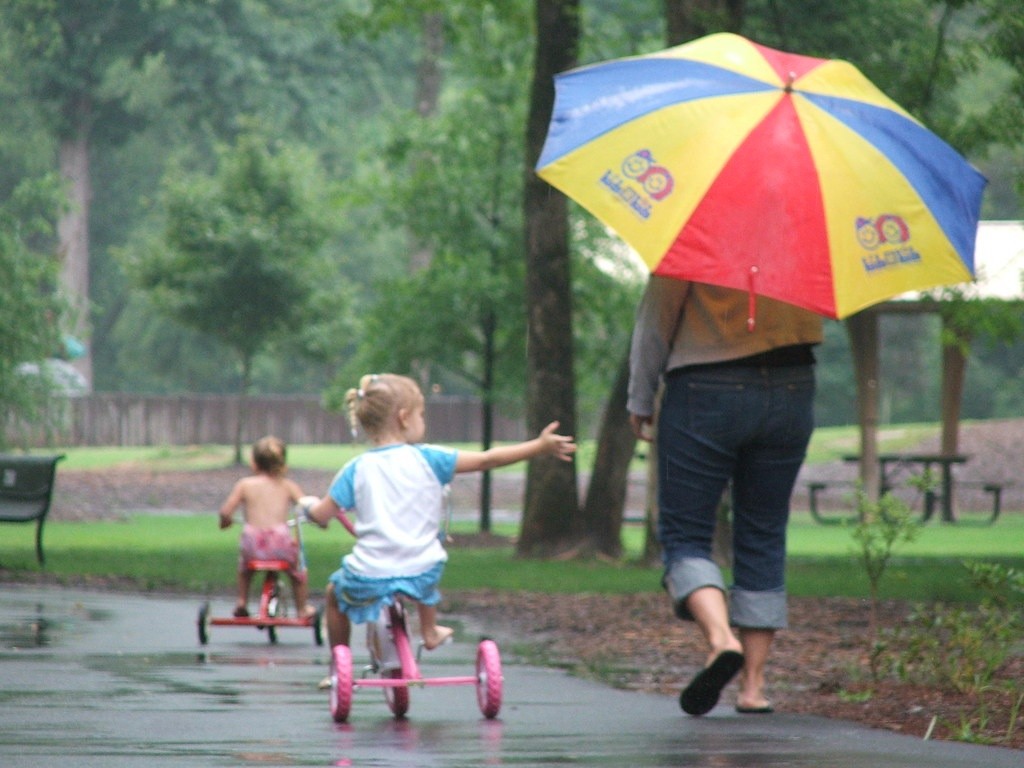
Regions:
[
  {"x1": 624, "y1": 272, "x2": 824, "y2": 714},
  {"x1": 218, "y1": 436, "x2": 315, "y2": 619},
  {"x1": 298, "y1": 373, "x2": 577, "y2": 690}
]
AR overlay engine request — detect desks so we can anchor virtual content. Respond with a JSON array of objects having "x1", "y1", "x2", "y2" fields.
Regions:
[{"x1": 841, "y1": 453, "x2": 972, "y2": 525}]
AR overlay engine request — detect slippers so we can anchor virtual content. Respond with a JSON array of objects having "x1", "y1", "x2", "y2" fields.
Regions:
[
  {"x1": 735, "y1": 696, "x2": 773, "y2": 712},
  {"x1": 680, "y1": 650, "x2": 745, "y2": 715}
]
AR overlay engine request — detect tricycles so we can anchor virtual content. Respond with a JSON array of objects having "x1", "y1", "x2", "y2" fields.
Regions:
[
  {"x1": 194, "y1": 513, "x2": 332, "y2": 645},
  {"x1": 282, "y1": 500, "x2": 505, "y2": 723}
]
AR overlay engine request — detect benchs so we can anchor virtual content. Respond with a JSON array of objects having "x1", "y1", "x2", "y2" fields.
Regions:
[
  {"x1": 954, "y1": 480, "x2": 1016, "y2": 525},
  {"x1": 800, "y1": 480, "x2": 854, "y2": 525},
  {"x1": 0, "y1": 453, "x2": 67, "y2": 567}
]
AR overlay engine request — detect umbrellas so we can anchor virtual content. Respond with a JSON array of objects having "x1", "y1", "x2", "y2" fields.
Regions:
[{"x1": 534, "y1": 30, "x2": 987, "y2": 333}]
